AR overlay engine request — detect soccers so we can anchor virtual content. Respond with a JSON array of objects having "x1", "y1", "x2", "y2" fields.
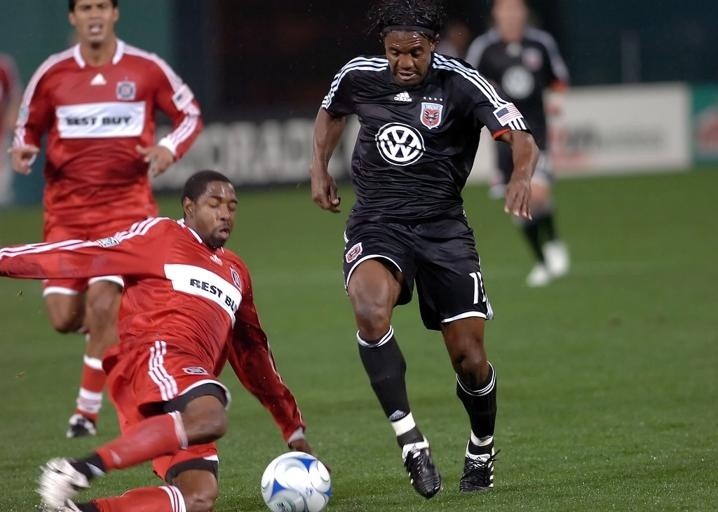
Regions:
[{"x1": 261, "y1": 451, "x2": 332, "y2": 512}]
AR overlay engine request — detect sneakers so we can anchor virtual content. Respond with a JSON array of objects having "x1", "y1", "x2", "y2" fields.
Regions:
[
  {"x1": 67, "y1": 413, "x2": 97, "y2": 437},
  {"x1": 459, "y1": 431, "x2": 500, "y2": 491},
  {"x1": 39, "y1": 458, "x2": 92, "y2": 512},
  {"x1": 400, "y1": 435, "x2": 440, "y2": 497}
]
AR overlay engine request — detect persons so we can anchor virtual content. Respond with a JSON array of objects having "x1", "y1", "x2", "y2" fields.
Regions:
[
  {"x1": 464, "y1": 0, "x2": 570, "y2": 287},
  {"x1": 0, "y1": 171, "x2": 330, "y2": 512},
  {"x1": 310, "y1": 0, "x2": 540, "y2": 499},
  {"x1": 6, "y1": 0, "x2": 204, "y2": 438},
  {"x1": 1, "y1": 54, "x2": 21, "y2": 178}
]
